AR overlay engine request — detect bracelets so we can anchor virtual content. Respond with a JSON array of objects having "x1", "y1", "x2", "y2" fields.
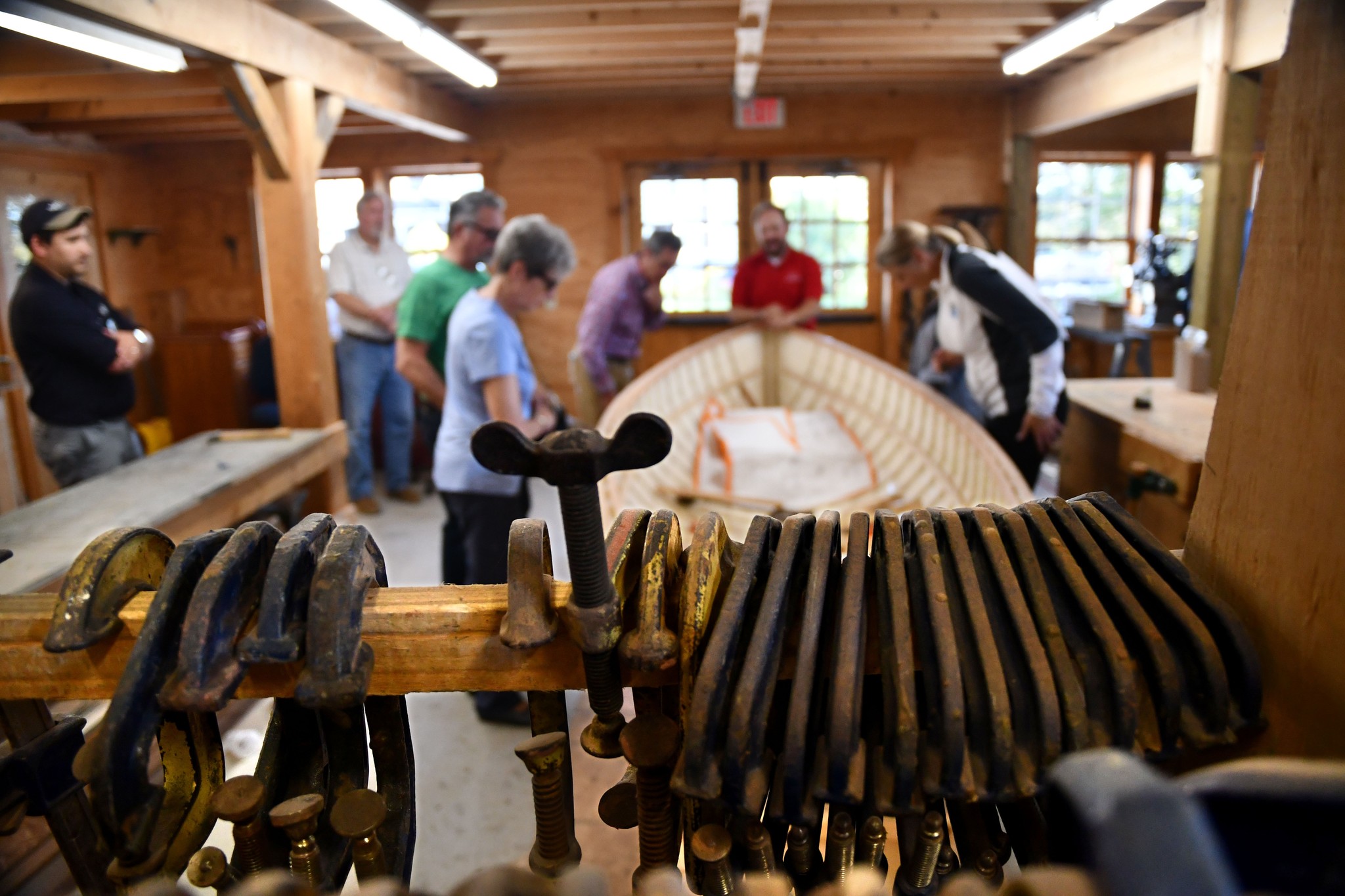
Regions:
[{"x1": 132, "y1": 327, "x2": 148, "y2": 358}]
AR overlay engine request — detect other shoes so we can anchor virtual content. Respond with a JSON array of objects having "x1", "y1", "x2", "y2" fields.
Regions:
[
  {"x1": 355, "y1": 495, "x2": 380, "y2": 514},
  {"x1": 495, "y1": 698, "x2": 530, "y2": 726},
  {"x1": 387, "y1": 485, "x2": 421, "y2": 504}
]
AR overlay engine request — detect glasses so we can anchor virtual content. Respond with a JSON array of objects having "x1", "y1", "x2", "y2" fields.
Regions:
[
  {"x1": 466, "y1": 220, "x2": 499, "y2": 240},
  {"x1": 535, "y1": 269, "x2": 557, "y2": 291}
]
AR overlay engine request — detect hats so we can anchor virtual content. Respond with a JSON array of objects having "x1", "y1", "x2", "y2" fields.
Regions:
[{"x1": 19, "y1": 198, "x2": 93, "y2": 245}]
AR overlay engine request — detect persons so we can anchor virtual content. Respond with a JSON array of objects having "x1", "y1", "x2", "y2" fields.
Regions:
[
  {"x1": 569, "y1": 230, "x2": 679, "y2": 429},
  {"x1": 873, "y1": 203, "x2": 1070, "y2": 497},
  {"x1": 8, "y1": 200, "x2": 159, "y2": 488},
  {"x1": 327, "y1": 191, "x2": 421, "y2": 515},
  {"x1": 433, "y1": 211, "x2": 575, "y2": 727},
  {"x1": 395, "y1": 191, "x2": 531, "y2": 585},
  {"x1": 731, "y1": 201, "x2": 824, "y2": 335}
]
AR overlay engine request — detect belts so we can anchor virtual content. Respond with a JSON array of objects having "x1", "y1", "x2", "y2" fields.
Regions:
[
  {"x1": 605, "y1": 354, "x2": 629, "y2": 365},
  {"x1": 342, "y1": 331, "x2": 394, "y2": 345}
]
